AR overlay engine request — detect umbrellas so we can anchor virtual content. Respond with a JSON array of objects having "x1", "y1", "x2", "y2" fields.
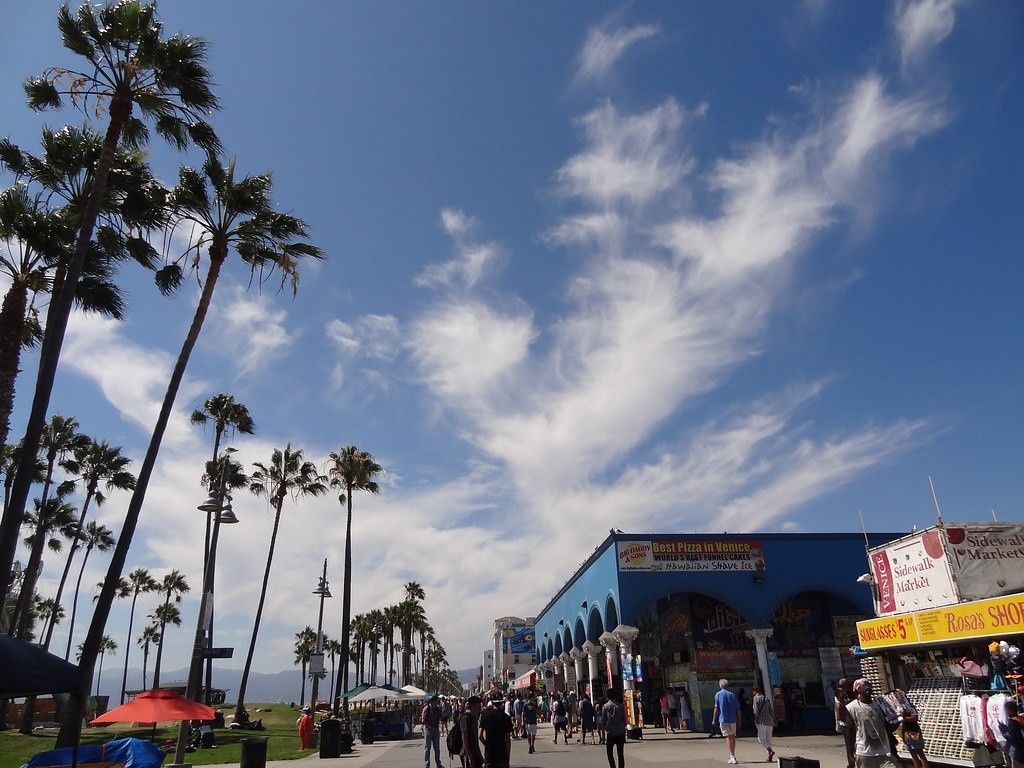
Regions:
[
  {"x1": 336, "y1": 682, "x2": 373, "y2": 700},
  {"x1": 347, "y1": 685, "x2": 399, "y2": 702},
  {"x1": 388, "y1": 685, "x2": 429, "y2": 700},
  {"x1": 89, "y1": 690, "x2": 217, "y2": 744},
  {"x1": 381, "y1": 684, "x2": 415, "y2": 694}
]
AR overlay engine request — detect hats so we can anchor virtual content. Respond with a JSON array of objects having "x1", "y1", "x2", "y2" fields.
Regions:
[
  {"x1": 490, "y1": 691, "x2": 505, "y2": 703},
  {"x1": 839, "y1": 679, "x2": 849, "y2": 686}
]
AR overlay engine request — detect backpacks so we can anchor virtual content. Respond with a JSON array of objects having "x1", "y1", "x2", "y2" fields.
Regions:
[{"x1": 446, "y1": 712, "x2": 467, "y2": 761}]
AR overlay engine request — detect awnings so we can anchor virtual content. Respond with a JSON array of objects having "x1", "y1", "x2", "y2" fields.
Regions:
[{"x1": 515, "y1": 670, "x2": 536, "y2": 689}]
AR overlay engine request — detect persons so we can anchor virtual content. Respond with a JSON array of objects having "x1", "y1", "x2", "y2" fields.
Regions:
[
  {"x1": 997, "y1": 701, "x2": 1024, "y2": 768},
  {"x1": 478, "y1": 691, "x2": 514, "y2": 768},
  {"x1": 458, "y1": 696, "x2": 485, "y2": 768},
  {"x1": 752, "y1": 686, "x2": 776, "y2": 763},
  {"x1": 834, "y1": 678, "x2": 929, "y2": 768},
  {"x1": 438, "y1": 689, "x2": 605, "y2": 745},
  {"x1": 660, "y1": 687, "x2": 693, "y2": 733},
  {"x1": 712, "y1": 679, "x2": 741, "y2": 764},
  {"x1": 602, "y1": 689, "x2": 626, "y2": 768},
  {"x1": 421, "y1": 696, "x2": 445, "y2": 768},
  {"x1": 521, "y1": 693, "x2": 541, "y2": 754},
  {"x1": 296, "y1": 714, "x2": 313, "y2": 750},
  {"x1": 550, "y1": 693, "x2": 568, "y2": 746}
]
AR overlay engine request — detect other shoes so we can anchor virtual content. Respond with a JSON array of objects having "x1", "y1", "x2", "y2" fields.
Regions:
[
  {"x1": 728, "y1": 756, "x2": 737, "y2": 764},
  {"x1": 766, "y1": 752, "x2": 775, "y2": 761},
  {"x1": 529, "y1": 746, "x2": 535, "y2": 754},
  {"x1": 564, "y1": 742, "x2": 568, "y2": 744},
  {"x1": 551, "y1": 740, "x2": 557, "y2": 744}
]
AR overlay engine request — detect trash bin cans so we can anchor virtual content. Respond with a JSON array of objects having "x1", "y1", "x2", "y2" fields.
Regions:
[
  {"x1": 319, "y1": 718, "x2": 342, "y2": 758},
  {"x1": 240, "y1": 735, "x2": 269, "y2": 768}
]
